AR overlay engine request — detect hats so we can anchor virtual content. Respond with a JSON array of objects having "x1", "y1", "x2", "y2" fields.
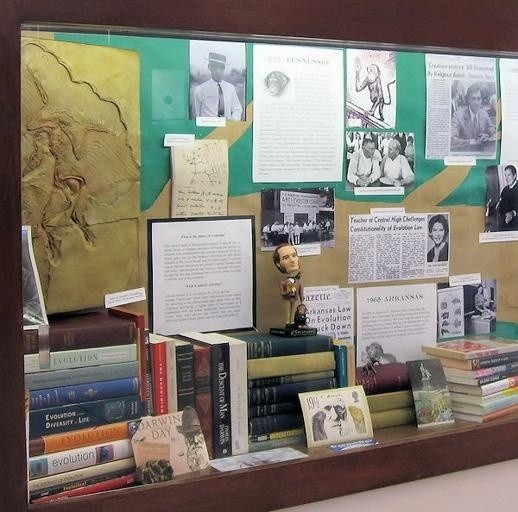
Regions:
[
  {"x1": 203, "y1": 53, "x2": 228, "y2": 65},
  {"x1": 176, "y1": 406, "x2": 200, "y2": 434}
]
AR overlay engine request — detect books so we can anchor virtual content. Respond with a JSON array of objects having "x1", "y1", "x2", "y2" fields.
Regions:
[
  {"x1": 23, "y1": 311, "x2": 249, "y2": 505},
  {"x1": 357, "y1": 334, "x2": 517, "y2": 430},
  {"x1": 229, "y1": 331, "x2": 356, "y2": 443}
]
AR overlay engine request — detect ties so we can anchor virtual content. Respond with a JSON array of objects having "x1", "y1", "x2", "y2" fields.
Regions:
[
  {"x1": 473, "y1": 115, "x2": 479, "y2": 138},
  {"x1": 218, "y1": 84, "x2": 225, "y2": 117}
]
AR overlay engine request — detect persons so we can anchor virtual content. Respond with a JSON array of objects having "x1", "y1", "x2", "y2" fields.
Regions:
[
  {"x1": 272, "y1": 243, "x2": 306, "y2": 329},
  {"x1": 488, "y1": 165, "x2": 518, "y2": 232},
  {"x1": 470, "y1": 284, "x2": 496, "y2": 322},
  {"x1": 427, "y1": 214, "x2": 450, "y2": 262},
  {"x1": 380, "y1": 140, "x2": 415, "y2": 187},
  {"x1": 263, "y1": 218, "x2": 331, "y2": 246},
  {"x1": 190, "y1": 53, "x2": 244, "y2": 121},
  {"x1": 346, "y1": 139, "x2": 383, "y2": 186},
  {"x1": 453, "y1": 85, "x2": 495, "y2": 151}
]
